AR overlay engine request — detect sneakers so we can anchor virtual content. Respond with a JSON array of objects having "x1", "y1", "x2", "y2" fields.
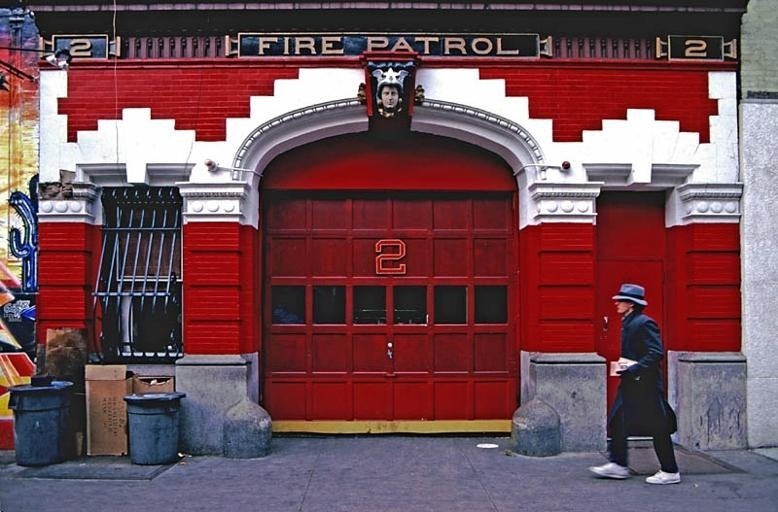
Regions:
[
  {"x1": 588, "y1": 461, "x2": 629, "y2": 480},
  {"x1": 645, "y1": 469, "x2": 681, "y2": 485}
]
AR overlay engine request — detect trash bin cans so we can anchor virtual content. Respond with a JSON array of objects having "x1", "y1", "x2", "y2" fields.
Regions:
[
  {"x1": 8, "y1": 380, "x2": 75, "y2": 467},
  {"x1": 123, "y1": 391, "x2": 186, "y2": 465}
]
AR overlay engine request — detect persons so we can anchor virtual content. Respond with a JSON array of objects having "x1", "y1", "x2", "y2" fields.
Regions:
[
  {"x1": 379, "y1": 73, "x2": 402, "y2": 118},
  {"x1": 589, "y1": 283, "x2": 684, "y2": 485}
]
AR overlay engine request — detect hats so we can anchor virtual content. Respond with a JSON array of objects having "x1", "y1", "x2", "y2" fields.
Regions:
[{"x1": 612, "y1": 284, "x2": 648, "y2": 307}]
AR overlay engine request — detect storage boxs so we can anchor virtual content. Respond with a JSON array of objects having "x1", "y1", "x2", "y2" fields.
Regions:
[{"x1": 82, "y1": 364, "x2": 176, "y2": 457}]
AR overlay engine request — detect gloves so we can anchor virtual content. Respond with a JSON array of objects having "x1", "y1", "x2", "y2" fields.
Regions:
[{"x1": 617, "y1": 369, "x2": 633, "y2": 383}]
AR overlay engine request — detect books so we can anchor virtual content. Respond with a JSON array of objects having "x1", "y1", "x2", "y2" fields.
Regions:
[{"x1": 609, "y1": 357, "x2": 640, "y2": 379}]
